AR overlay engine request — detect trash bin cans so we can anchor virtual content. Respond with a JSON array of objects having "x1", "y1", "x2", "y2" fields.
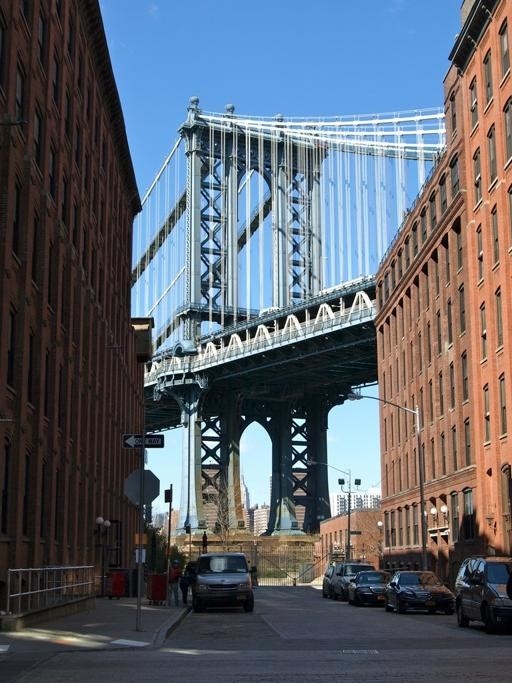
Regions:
[
  {"x1": 106, "y1": 571, "x2": 128, "y2": 600},
  {"x1": 147, "y1": 574, "x2": 166, "y2": 606}
]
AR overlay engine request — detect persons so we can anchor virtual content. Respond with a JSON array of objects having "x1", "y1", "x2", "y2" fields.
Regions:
[
  {"x1": 167, "y1": 558, "x2": 183, "y2": 608},
  {"x1": 185, "y1": 560, "x2": 194, "y2": 575},
  {"x1": 179, "y1": 576, "x2": 189, "y2": 604}
]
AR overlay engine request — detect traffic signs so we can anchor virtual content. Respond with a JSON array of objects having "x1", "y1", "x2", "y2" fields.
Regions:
[{"x1": 121, "y1": 434, "x2": 164, "y2": 449}]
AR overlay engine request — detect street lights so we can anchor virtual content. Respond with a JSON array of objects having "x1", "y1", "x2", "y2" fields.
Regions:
[
  {"x1": 347, "y1": 392, "x2": 428, "y2": 571},
  {"x1": 307, "y1": 460, "x2": 362, "y2": 561},
  {"x1": 96, "y1": 516, "x2": 111, "y2": 598}
]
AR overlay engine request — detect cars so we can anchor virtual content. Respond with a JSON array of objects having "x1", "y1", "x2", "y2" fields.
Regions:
[
  {"x1": 194, "y1": 552, "x2": 257, "y2": 612},
  {"x1": 323, "y1": 556, "x2": 512, "y2": 634}
]
